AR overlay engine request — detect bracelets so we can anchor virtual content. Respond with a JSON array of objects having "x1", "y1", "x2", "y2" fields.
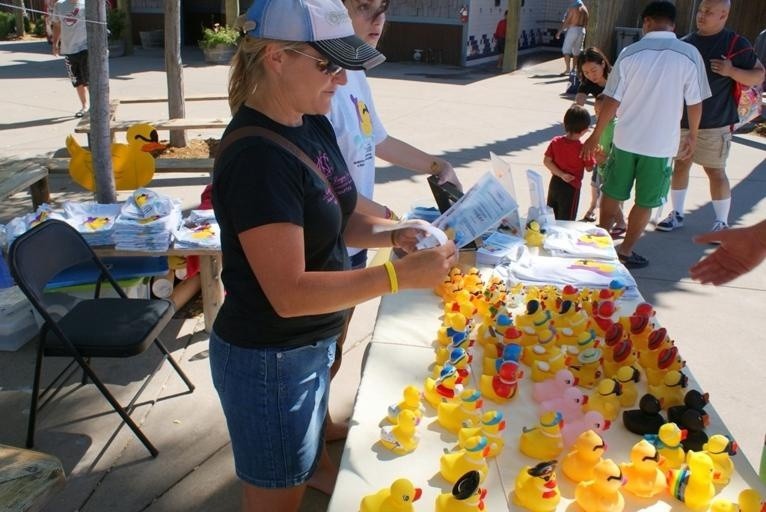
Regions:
[
  {"x1": 384, "y1": 260, "x2": 399, "y2": 295},
  {"x1": 383, "y1": 205, "x2": 398, "y2": 222}
]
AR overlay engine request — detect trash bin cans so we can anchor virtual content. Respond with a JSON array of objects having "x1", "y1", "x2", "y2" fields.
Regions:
[{"x1": 614, "y1": 25, "x2": 643, "y2": 59}]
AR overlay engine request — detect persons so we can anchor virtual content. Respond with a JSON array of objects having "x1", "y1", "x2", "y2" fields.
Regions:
[
  {"x1": 493, "y1": 10, "x2": 509, "y2": 69},
  {"x1": 654, "y1": 0, "x2": 765, "y2": 245},
  {"x1": 686, "y1": 218, "x2": 765, "y2": 287},
  {"x1": 571, "y1": 46, "x2": 627, "y2": 238},
  {"x1": 577, "y1": 0, "x2": 713, "y2": 269},
  {"x1": 51, "y1": 0, "x2": 91, "y2": 117},
  {"x1": 305, "y1": 0, "x2": 465, "y2": 497},
  {"x1": 554, "y1": 0, "x2": 590, "y2": 77},
  {"x1": 206, "y1": 0, "x2": 460, "y2": 511},
  {"x1": 542, "y1": 102, "x2": 604, "y2": 222}
]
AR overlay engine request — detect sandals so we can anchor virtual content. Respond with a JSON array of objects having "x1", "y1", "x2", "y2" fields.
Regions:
[{"x1": 76, "y1": 108, "x2": 86, "y2": 117}]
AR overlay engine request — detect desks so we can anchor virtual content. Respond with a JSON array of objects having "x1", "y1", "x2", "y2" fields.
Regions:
[
  {"x1": 321, "y1": 218, "x2": 762, "y2": 512},
  {"x1": 91, "y1": 213, "x2": 225, "y2": 335}
]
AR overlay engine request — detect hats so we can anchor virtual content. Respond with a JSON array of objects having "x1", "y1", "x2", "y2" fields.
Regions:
[{"x1": 240, "y1": 0, "x2": 386, "y2": 69}]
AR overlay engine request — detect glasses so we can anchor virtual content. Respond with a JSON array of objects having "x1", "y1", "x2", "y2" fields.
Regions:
[{"x1": 285, "y1": 44, "x2": 343, "y2": 75}]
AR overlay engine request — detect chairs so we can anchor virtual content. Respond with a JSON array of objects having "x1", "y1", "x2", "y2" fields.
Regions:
[{"x1": 8, "y1": 218, "x2": 194, "y2": 458}]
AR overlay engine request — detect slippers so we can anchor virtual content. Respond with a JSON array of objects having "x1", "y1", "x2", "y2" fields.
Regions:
[{"x1": 610, "y1": 227, "x2": 626, "y2": 238}]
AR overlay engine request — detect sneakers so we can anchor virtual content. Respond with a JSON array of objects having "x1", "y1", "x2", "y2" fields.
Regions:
[
  {"x1": 655, "y1": 211, "x2": 684, "y2": 231},
  {"x1": 618, "y1": 252, "x2": 649, "y2": 268},
  {"x1": 710, "y1": 220, "x2": 728, "y2": 244}
]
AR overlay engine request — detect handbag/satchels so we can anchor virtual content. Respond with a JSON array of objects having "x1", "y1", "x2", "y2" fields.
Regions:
[{"x1": 731, "y1": 78, "x2": 762, "y2": 133}]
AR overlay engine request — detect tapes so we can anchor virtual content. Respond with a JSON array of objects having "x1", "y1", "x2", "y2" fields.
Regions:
[{"x1": 372, "y1": 222, "x2": 448, "y2": 258}]
